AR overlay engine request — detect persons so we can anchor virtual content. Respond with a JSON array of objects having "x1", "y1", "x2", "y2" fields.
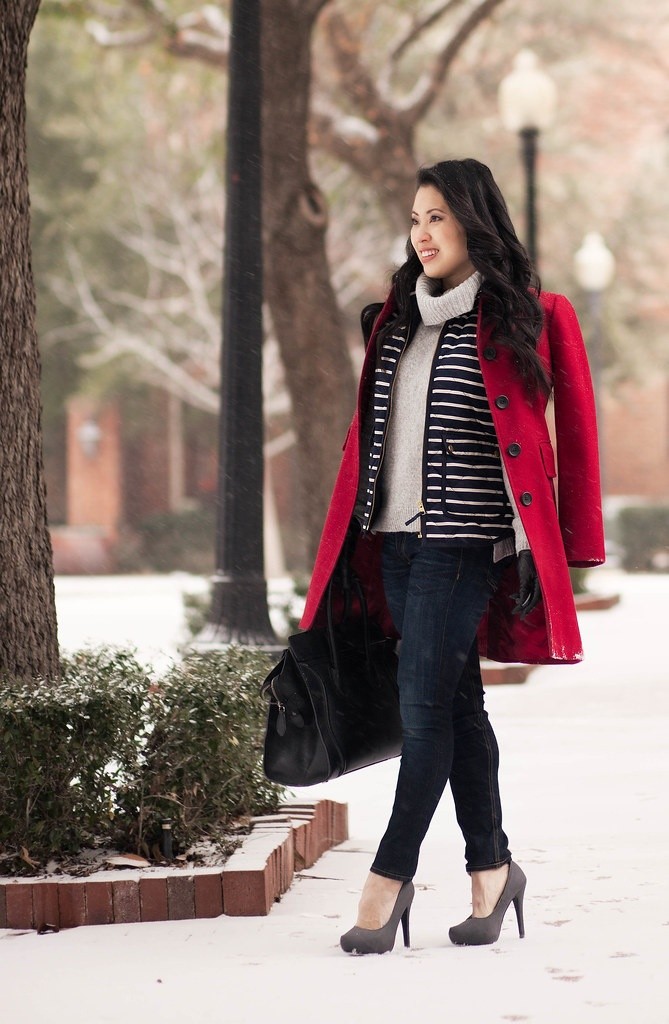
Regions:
[{"x1": 298, "y1": 157, "x2": 606, "y2": 955}]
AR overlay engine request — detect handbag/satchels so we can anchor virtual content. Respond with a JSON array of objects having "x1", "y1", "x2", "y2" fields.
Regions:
[{"x1": 261, "y1": 570, "x2": 409, "y2": 787}]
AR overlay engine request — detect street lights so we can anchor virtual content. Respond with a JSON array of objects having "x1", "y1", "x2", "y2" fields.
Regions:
[
  {"x1": 571, "y1": 228, "x2": 615, "y2": 472},
  {"x1": 499, "y1": 44, "x2": 558, "y2": 291}
]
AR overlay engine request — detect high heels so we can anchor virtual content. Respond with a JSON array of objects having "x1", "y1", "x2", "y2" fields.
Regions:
[
  {"x1": 340, "y1": 879, "x2": 414, "y2": 956},
  {"x1": 448, "y1": 859, "x2": 527, "y2": 946}
]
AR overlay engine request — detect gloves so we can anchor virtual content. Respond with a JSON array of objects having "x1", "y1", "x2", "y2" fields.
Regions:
[
  {"x1": 508, "y1": 551, "x2": 540, "y2": 620},
  {"x1": 331, "y1": 519, "x2": 365, "y2": 595}
]
